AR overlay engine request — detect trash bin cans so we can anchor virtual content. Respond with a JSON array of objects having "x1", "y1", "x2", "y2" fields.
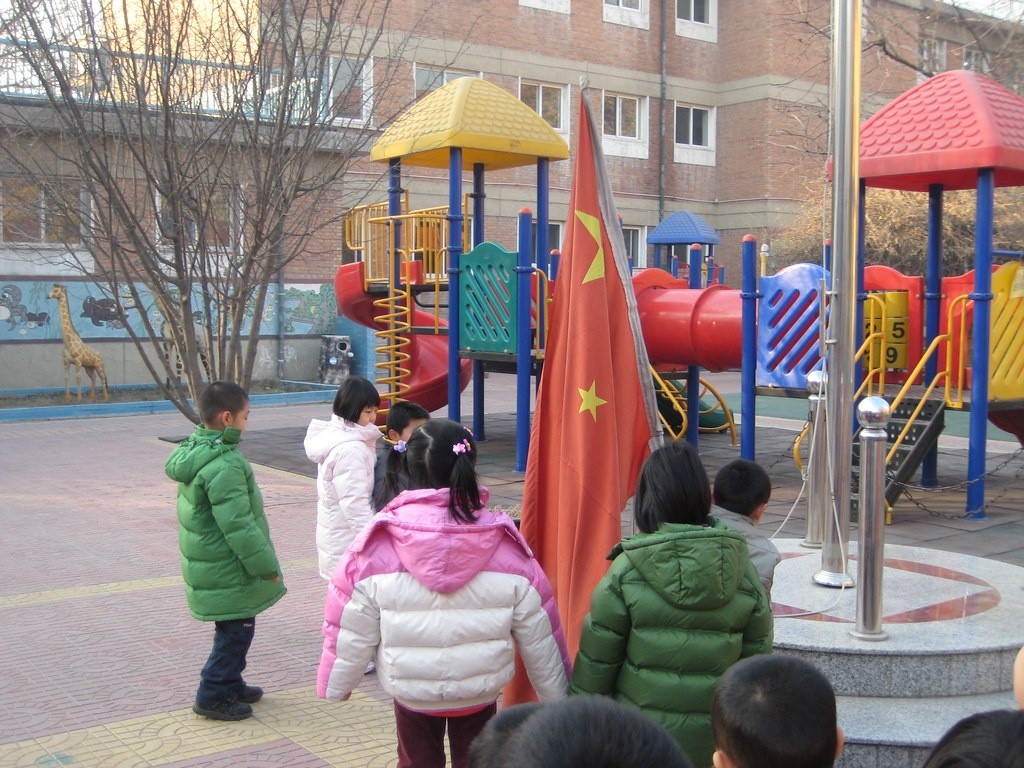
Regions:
[{"x1": 318, "y1": 335, "x2": 352, "y2": 385}]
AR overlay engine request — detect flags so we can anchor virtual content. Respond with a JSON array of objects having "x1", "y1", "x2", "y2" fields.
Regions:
[{"x1": 503, "y1": 92, "x2": 660, "y2": 709}]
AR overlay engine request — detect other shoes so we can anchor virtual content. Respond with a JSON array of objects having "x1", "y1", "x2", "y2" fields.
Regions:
[{"x1": 364, "y1": 653, "x2": 375, "y2": 673}]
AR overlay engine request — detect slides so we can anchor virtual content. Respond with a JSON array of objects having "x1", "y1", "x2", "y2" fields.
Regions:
[{"x1": 332, "y1": 258, "x2": 474, "y2": 427}]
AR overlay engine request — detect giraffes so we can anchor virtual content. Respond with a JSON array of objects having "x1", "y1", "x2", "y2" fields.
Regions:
[
  {"x1": 135, "y1": 247, "x2": 213, "y2": 398},
  {"x1": 47, "y1": 284, "x2": 110, "y2": 402}
]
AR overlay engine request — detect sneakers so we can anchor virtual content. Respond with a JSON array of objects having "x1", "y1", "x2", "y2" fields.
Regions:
[
  {"x1": 236, "y1": 682, "x2": 264, "y2": 703},
  {"x1": 192, "y1": 695, "x2": 252, "y2": 720}
]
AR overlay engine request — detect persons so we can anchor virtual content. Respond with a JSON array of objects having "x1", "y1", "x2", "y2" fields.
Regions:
[
  {"x1": 374, "y1": 401, "x2": 430, "y2": 512},
  {"x1": 316, "y1": 418, "x2": 572, "y2": 768},
  {"x1": 922, "y1": 646, "x2": 1024, "y2": 768},
  {"x1": 466, "y1": 695, "x2": 690, "y2": 768},
  {"x1": 568, "y1": 444, "x2": 774, "y2": 768},
  {"x1": 709, "y1": 458, "x2": 781, "y2": 610},
  {"x1": 713, "y1": 655, "x2": 844, "y2": 768},
  {"x1": 304, "y1": 377, "x2": 385, "y2": 581},
  {"x1": 165, "y1": 380, "x2": 287, "y2": 720}
]
